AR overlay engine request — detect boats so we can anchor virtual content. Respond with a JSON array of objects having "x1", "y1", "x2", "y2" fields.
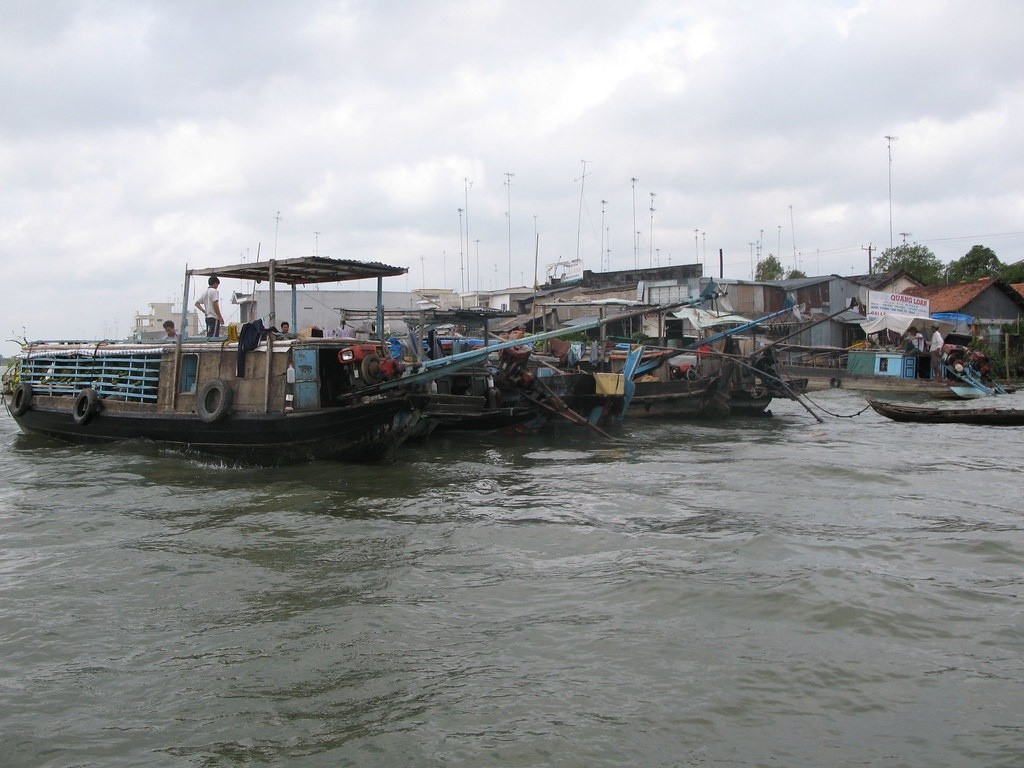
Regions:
[
  {"x1": 866, "y1": 398, "x2": 1023, "y2": 428},
  {"x1": 638, "y1": 304, "x2": 858, "y2": 415},
  {"x1": 8, "y1": 254, "x2": 413, "y2": 467},
  {"x1": 407, "y1": 306, "x2": 634, "y2": 430}
]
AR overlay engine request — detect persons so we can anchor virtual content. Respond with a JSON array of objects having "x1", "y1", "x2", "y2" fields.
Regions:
[
  {"x1": 898, "y1": 326, "x2": 924, "y2": 355},
  {"x1": 194, "y1": 276, "x2": 224, "y2": 337},
  {"x1": 162, "y1": 320, "x2": 180, "y2": 340},
  {"x1": 281, "y1": 322, "x2": 289, "y2": 334},
  {"x1": 929, "y1": 323, "x2": 945, "y2": 379}
]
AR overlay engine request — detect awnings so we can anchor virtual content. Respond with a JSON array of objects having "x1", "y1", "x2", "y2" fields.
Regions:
[{"x1": 860, "y1": 313, "x2": 955, "y2": 345}]
